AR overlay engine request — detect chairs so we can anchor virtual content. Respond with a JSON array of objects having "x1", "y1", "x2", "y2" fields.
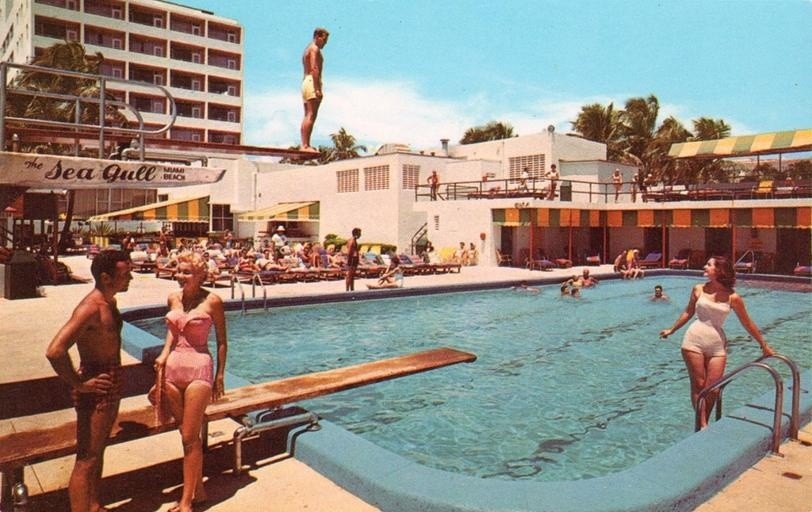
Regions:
[{"x1": 641, "y1": 179, "x2": 812, "y2": 202}]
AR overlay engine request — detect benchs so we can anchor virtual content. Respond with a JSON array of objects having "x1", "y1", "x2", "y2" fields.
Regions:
[{"x1": 467, "y1": 188, "x2": 562, "y2": 203}]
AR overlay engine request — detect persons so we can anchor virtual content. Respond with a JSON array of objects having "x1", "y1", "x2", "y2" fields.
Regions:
[
  {"x1": 451, "y1": 241, "x2": 467, "y2": 266},
  {"x1": 44, "y1": 250, "x2": 134, "y2": 512},
  {"x1": 465, "y1": 242, "x2": 478, "y2": 266},
  {"x1": 345, "y1": 227, "x2": 362, "y2": 291},
  {"x1": 613, "y1": 248, "x2": 631, "y2": 282},
  {"x1": 629, "y1": 173, "x2": 640, "y2": 202},
  {"x1": 521, "y1": 167, "x2": 530, "y2": 192},
  {"x1": 544, "y1": 163, "x2": 560, "y2": 200},
  {"x1": 626, "y1": 249, "x2": 645, "y2": 280},
  {"x1": 297, "y1": 25, "x2": 329, "y2": 155},
  {"x1": 658, "y1": 254, "x2": 775, "y2": 434},
  {"x1": 559, "y1": 269, "x2": 600, "y2": 298},
  {"x1": 639, "y1": 173, "x2": 653, "y2": 202},
  {"x1": 649, "y1": 285, "x2": 670, "y2": 304},
  {"x1": 511, "y1": 279, "x2": 540, "y2": 294},
  {"x1": 611, "y1": 168, "x2": 624, "y2": 202},
  {"x1": 150, "y1": 252, "x2": 228, "y2": 512},
  {"x1": 427, "y1": 170, "x2": 439, "y2": 200},
  {"x1": 111, "y1": 225, "x2": 366, "y2": 284},
  {"x1": 365, "y1": 256, "x2": 404, "y2": 290}
]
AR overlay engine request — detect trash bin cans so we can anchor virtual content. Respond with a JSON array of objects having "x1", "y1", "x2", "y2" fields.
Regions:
[
  {"x1": 4, "y1": 251, "x2": 36, "y2": 299},
  {"x1": 560, "y1": 180, "x2": 572, "y2": 201},
  {"x1": 416, "y1": 240, "x2": 428, "y2": 255}
]
